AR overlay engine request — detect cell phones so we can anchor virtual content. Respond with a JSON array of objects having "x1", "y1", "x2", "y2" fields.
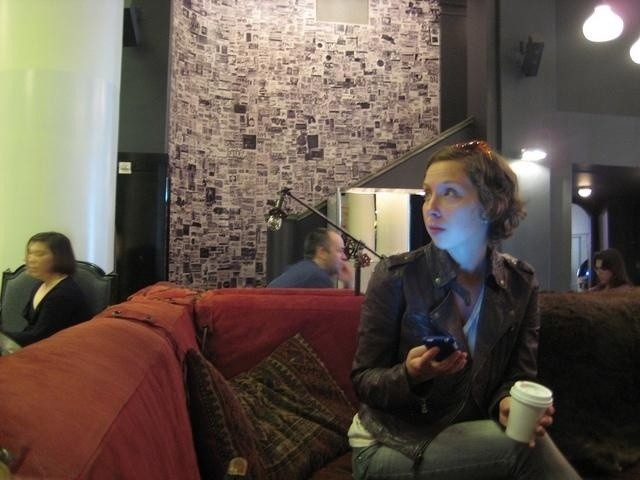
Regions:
[{"x1": 420, "y1": 335, "x2": 460, "y2": 364}]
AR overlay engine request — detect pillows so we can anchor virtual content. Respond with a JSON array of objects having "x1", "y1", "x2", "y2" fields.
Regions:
[{"x1": 180, "y1": 332, "x2": 362, "y2": 480}]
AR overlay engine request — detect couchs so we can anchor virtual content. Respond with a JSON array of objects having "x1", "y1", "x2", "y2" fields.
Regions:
[{"x1": 1, "y1": 281, "x2": 640, "y2": 480}]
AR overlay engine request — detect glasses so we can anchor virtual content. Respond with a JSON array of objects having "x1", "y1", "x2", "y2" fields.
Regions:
[{"x1": 451, "y1": 140, "x2": 514, "y2": 185}]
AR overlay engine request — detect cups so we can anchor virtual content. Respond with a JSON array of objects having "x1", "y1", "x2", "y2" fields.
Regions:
[{"x1": 505, "y1": 378, "x2": 554, "y2": 446}]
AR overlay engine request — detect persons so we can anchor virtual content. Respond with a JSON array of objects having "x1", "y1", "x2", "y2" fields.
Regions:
[
  {"x1": 4, "y1": 229, "x2": 89, "y2": 347},
  {"x1": 265, "y1": 226, "x2": 356, "y2": 289},
  {"x1": 345, "y1": 137, "x2": 585, "y2": 479},
  {"x1": 575, "y1": 247, "x2": 632, "y2": 293}
]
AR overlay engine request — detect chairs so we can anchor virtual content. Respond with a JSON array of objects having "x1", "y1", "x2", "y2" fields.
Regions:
[{"x1": 0, "y1": 261, "x2": 123, "y2": 340}]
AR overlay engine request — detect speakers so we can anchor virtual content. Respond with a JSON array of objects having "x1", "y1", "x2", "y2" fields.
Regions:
[{"x1": 521, "y1": 31, "x2": 543, "y2": 76}]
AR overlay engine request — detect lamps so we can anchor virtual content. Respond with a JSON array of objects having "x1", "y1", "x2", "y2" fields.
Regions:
[
  {"x1": 582, "y1": 0, "x2": 624, "y2": 43},
  {"x1": 629, "y1": 36, "x2": 640, "y2": 66},
  {"x1": 575, "y1": 171, "x2": 594, "y2": 198},
  {"x1": 265, "y1": 187, "x2": 385, "y2": 295}
]
